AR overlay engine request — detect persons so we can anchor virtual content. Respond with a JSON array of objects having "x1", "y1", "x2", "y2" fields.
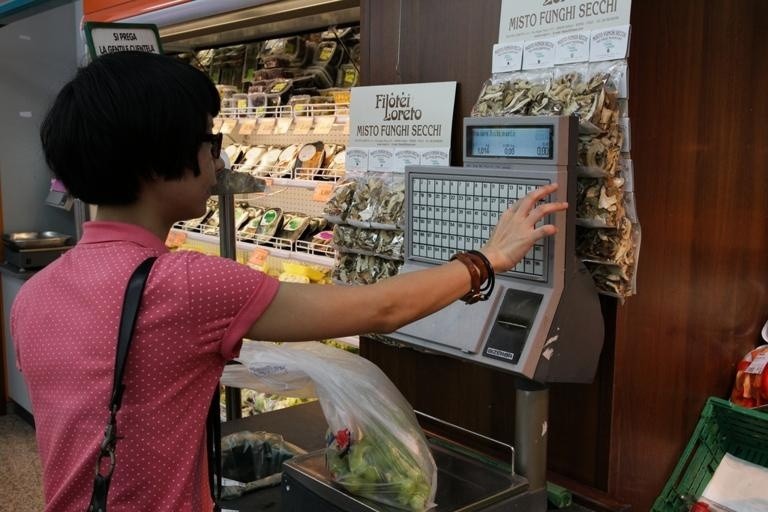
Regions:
[{"x1": 7, "y1": 50, "x2": 573, "y2": 511}]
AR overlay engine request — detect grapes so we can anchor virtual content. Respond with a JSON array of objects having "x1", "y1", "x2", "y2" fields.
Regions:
[{"x1": 324, "y1": 422, "x2": 430, "y2": 510}]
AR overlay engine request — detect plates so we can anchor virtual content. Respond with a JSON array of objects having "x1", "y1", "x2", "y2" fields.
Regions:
[{"x1": 186, "y1": 27, "x2": 361, "y2": 257}]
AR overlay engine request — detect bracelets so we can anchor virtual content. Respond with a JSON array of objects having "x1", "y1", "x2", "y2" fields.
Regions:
[{"x1": 446, "y1": 250, "x2": 495, "y2": 303}]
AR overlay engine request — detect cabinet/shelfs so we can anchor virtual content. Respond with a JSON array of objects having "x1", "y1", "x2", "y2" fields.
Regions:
[{"x1": 160, "y1": 20, "x2": 365, "y2": 354}]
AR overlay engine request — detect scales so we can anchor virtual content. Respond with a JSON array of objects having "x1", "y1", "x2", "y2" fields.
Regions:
[
  {"x1": 281, "y1": 115, "x2": 606, "y2": 512},
  {"x1": 0, "y1": 180, "x2": 88, "y2": 273}
]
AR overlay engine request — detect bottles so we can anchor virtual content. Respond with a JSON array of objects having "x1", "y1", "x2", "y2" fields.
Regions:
[{"x1": 673, "y1": 492, "x2": 736, "y2": 512}]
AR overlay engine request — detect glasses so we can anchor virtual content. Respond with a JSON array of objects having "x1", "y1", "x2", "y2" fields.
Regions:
[{"x1": 203, "y1": 130, "x2": 223, "y2": 159}]
